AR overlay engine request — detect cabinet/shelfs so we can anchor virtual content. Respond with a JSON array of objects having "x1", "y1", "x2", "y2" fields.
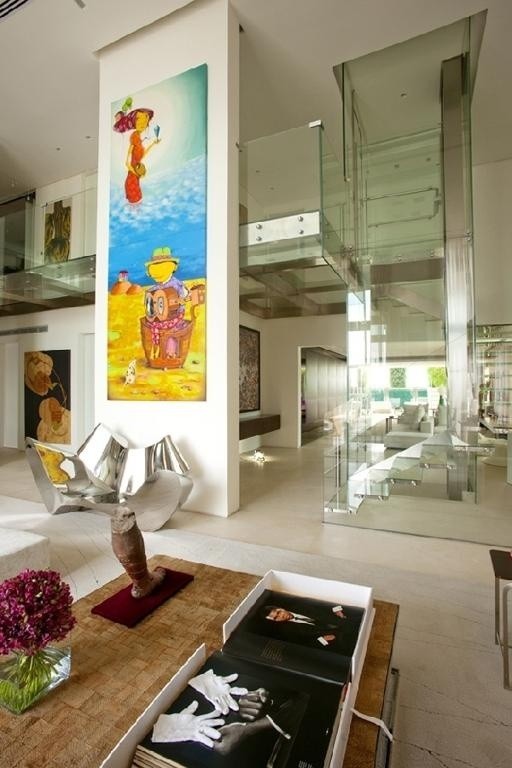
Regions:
[{"x1": 239, "y1": 413, "x2": 280, "y2": 440}]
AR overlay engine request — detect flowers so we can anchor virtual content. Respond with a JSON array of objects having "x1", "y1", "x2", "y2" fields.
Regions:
[{"x1": 0, "y1": 567, "x2": 76, "y2": 716}]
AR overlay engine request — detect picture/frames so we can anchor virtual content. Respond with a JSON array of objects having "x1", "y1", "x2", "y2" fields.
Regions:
[{"x1": 239, "y1": 323, "x2": 261, "y2": 414}]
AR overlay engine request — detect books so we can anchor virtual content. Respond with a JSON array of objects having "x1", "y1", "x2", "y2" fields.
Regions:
[{"x1": 130, "y1": 589, "x2": 367, "y2": 767}]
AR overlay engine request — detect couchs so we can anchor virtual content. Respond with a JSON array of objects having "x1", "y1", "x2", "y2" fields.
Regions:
[{"x1": 384, "y1": 404, "x2": 448, "y2": 453}]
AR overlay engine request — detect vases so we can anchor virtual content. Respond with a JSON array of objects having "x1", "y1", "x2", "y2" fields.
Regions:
[{"x1": 0, "y1": 645, "x2": 72, "y2": 715}]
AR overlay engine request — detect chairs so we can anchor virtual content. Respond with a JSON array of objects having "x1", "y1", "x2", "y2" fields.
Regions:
[{"x1": 24, "y1": 423, "x2": 194, "y2": 531}]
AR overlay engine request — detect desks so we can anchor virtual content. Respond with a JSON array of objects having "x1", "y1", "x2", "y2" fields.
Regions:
[{"x1": 0, "y1": 554, "x2": 403, "y2": 767}]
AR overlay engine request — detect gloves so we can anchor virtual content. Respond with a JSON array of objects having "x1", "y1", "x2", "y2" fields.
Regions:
[
  {"x1": 188, "y1": 669, "x2": 248, "y2": 715},
  {"x1": 151, "y1": 699, "x2": 225, "y2": 749}
]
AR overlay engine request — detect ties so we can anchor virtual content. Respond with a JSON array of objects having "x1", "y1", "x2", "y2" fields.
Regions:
[{"x1": 293, "y1": 617, "x2": 318, "y2": 624}]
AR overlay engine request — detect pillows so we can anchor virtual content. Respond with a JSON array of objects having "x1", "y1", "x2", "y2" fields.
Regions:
[{"x1": 398, "y1": 403, "x2": 430, "y2": 427}]
